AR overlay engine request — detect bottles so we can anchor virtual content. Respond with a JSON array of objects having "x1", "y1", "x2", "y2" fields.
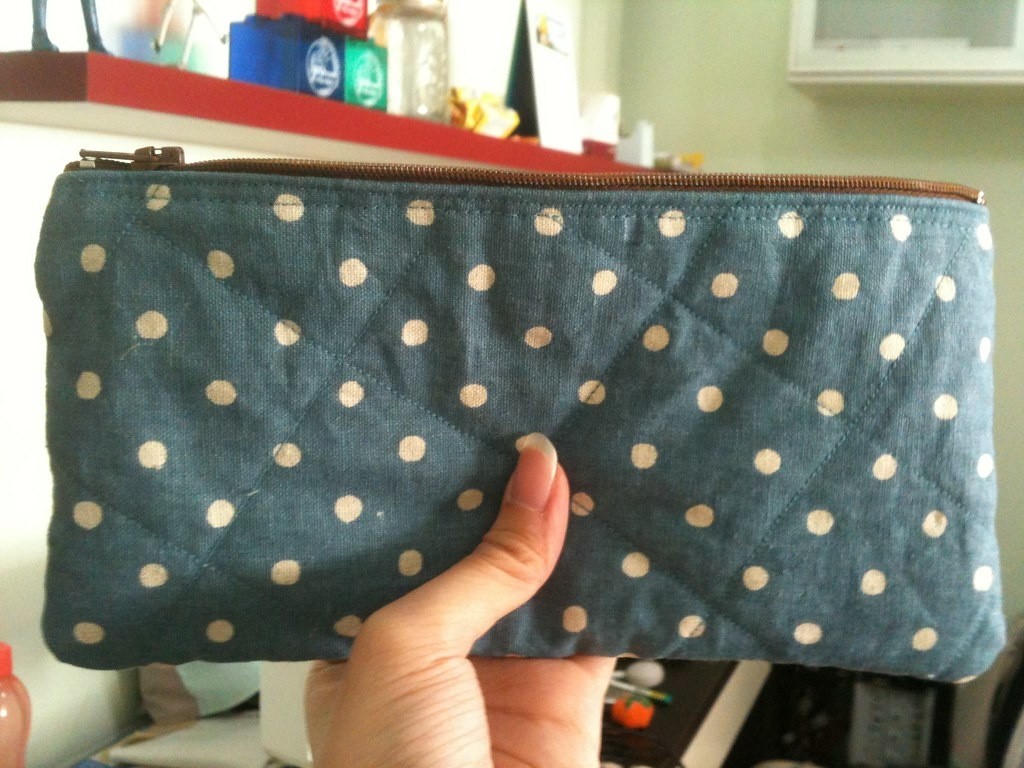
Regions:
[{"x1": 366, "y1": 1, "x2": 451, "y2": 127}]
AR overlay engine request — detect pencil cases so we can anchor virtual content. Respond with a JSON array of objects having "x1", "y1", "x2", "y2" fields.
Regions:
[{"x1": 38, "y1": 146, "x2": 1013, "y2": 688}]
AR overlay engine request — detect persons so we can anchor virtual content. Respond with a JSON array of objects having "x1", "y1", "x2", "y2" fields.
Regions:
[{"x1": 304, "y1": 430, "x2": 616, "y2": 768}]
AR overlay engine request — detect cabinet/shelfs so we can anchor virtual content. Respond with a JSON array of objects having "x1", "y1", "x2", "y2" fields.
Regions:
[
  {"x1": 784, "y1": 1, "x2": 1022, "y2": 101},
  {"x1": 3, "y1": 49, "x2": 689, "y2": 184}
]
